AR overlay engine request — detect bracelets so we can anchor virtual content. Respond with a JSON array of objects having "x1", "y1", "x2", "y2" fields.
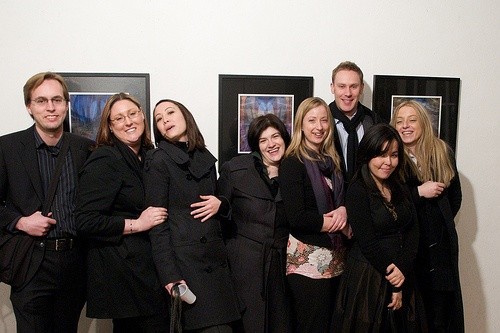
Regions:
[{"x1": 130, "y1": 219, "x2": 133, "y2": 234}]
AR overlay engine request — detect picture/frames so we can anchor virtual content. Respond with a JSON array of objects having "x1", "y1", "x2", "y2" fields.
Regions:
[
  {"x1": 372, "y1": 74, "x2": 460, "y2": 155},
  {"x1": 218, "y1": 74, "x2": 314, "y2": 174},
  {"x1": 55, "y1": 73, "x2": 151, "y2": 145}
]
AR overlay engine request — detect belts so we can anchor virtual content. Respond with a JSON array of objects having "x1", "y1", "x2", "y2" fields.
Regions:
[{"x1": 44, "y1": 238, "x2": 77, "y2": 251}]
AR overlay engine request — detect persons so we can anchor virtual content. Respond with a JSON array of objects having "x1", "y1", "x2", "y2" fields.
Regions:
[
  {"x1": 144, "y1": 100, "x2": 248, "y2": 333},
  {"x1": 74, "y1": 92, "x2": 171, "y2": 333},
  {"x1": 218, "y1": 113, "x2": 292, "y2": 333},
  {"x1": 335, "y1": 122, "x2": 430, "y2": 333},
  {"x1": 328, "y1": 61, "x2": 387, "y2": 171},
  {"x1": 277, "y1": 96, "x2": 354, "y2": 333},
  {"x1": 389, "y1": 100, "x2": 464, "y2": 333},
  {"x1": 0, "y1": 71, "x2": 95, "y2": 333}
]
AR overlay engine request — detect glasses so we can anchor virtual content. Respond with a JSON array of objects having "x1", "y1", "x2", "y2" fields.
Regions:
[
  {"x1": 109, "y1": 109, "x2": 141, "y2": 124},
  {"x1": 31, "y1": 96, "x2": 69, "y2": 106}
]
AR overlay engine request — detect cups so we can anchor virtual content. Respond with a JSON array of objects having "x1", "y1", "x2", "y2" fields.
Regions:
[{"x1": 174, "y1": 284, "x2": 196, "y2": 304}]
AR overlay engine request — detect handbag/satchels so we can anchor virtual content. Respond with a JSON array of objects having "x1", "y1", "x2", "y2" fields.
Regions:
[{"x1": 0, "y1": 229, "x2": 36, "y2": 287}]
